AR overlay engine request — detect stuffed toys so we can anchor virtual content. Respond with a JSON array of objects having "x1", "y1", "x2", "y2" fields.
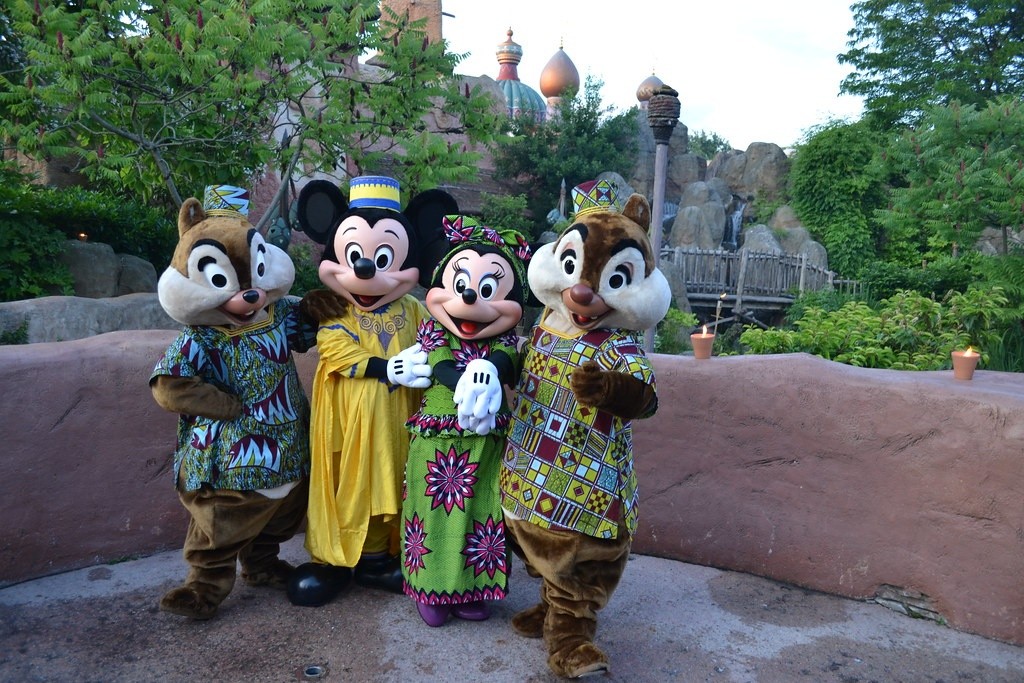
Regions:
[
  {"x1": 287, "y1": 176, "x2": 460, "y2": 608},
  {"x1": 499, "y1": 176, "x2": 673, "y2": 678},
  {"x1": 399, "y1": 215, "x2": 546, "y2": 628},
  {"x1": 148, "y1": 197, "x2": 349, "y2": 617}
]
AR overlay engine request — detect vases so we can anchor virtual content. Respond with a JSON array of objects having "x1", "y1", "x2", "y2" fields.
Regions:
[
  {"x1": 79, "y1": 234, "x2": 87, "y2": 243},
  {"x1": 951, "y1": 350, "x2": 980, "y2": 380},
  {"x1": 690, "y1": 333, "x2": 715, "y2": 359}
]
